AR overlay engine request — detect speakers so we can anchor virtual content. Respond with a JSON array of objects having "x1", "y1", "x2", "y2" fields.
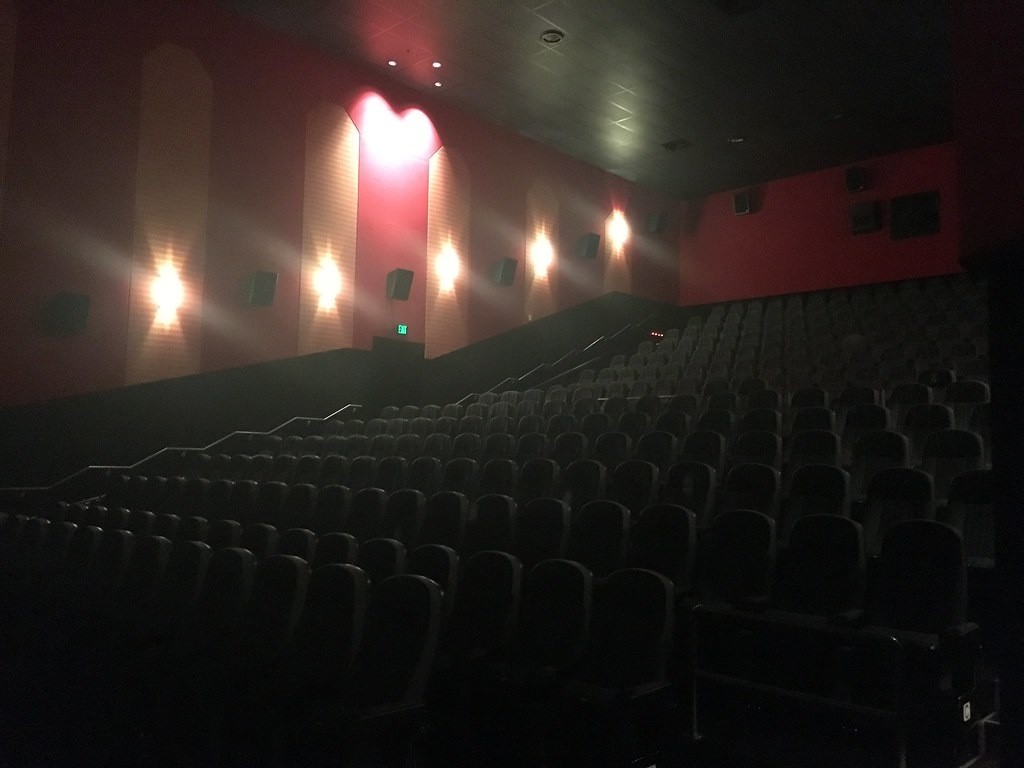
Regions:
[
  {"x1": 843, "y1": 167, "x2": 866, "y2": 194},
  {"x1": 386, "y1": 269, "x2": 414, "y2": 301},
  {"x1": 240, "y1": 273, "x2": 279, "y2": 310},
  {"x1": 491, "y1": 257, "x2": 517, "y2": 287},
  {"x1": 733, "y1": 190, "x2": 751, "y2": 216},
  {"x1": 43, "y1": 291, "x2": 90, "y2": 338},
  {"x1": 577, "y1": 233, "x2": 601, "y2": 258},
  {"x1": 645, "y1": 213, "x2": 664, "y2": 240}
]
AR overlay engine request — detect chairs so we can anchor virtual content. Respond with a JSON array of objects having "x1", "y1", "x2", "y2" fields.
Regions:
[{"x1": 0, "y1": 278, "x2": 1024, "y2": 768}]
